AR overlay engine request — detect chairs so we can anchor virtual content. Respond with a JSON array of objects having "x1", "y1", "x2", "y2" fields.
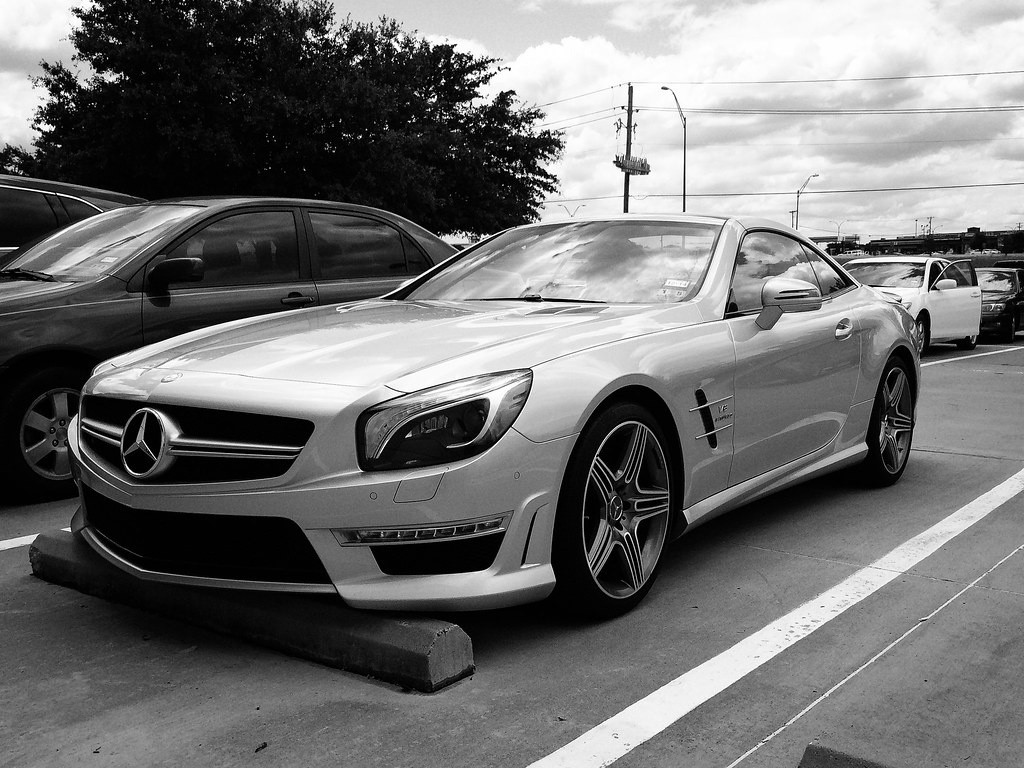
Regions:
[{"x1": 200, "y1": 236, "x2": 241, "y2": 283}]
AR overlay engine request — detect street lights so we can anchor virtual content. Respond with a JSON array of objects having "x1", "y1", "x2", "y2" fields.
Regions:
[
  {"x1": 661, "y1": 86, "x2": 686, "y2": 212},
  {"x1": 796, "y1": 173, "x2": 819, "y2": 230},
  {"x1": 829, "y1": 219, "x2": 850, "y2": 236}
]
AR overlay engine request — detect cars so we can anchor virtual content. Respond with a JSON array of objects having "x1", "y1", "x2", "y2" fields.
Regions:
[{"x1": 0, "y1": 174, "x2": 1024, "y2": 619}]
